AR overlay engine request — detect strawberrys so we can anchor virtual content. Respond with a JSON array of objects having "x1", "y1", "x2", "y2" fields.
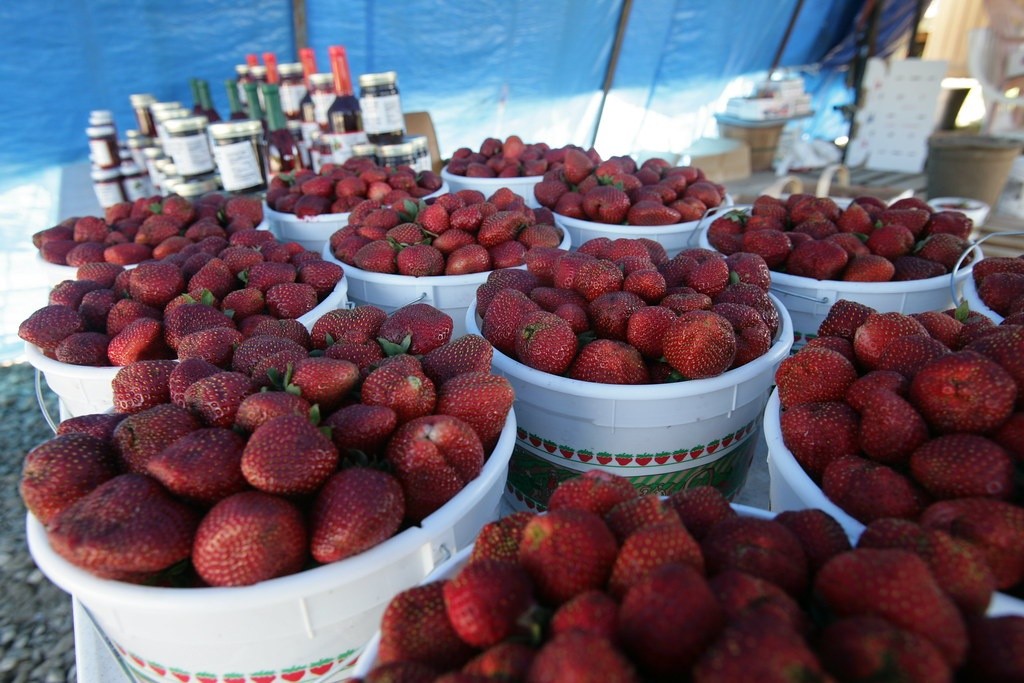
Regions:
[
  {"x1": 16, "y1": 134, "x2": 729, "y2": 586},
  {"x1": 360, "y1": 195, "x2": 1024, "y2": 683}
]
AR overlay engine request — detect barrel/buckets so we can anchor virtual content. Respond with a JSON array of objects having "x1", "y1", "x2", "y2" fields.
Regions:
[
  {"x1": 21, "y1": 274, "x2": 355, "y2": 436},
  {"x1": 462, "y1": 259, "x2": 794, "y2": 522},
  {"x1": 762, "y1": 382, "x2": 1023, "y2": 618},
  {"x1": 537, "y1": 194, "x2": 733, "y2": 261},
  {"x1": 717, "y1": 123, "x2": 786, "y2": 171},
  {"x1": 950, "y1": 230, "x2": 1023, "y2": 327},
  {"x1": 927, "y1": 199, "x2": 989, "y2": 242},
  {"x1": 320, "y1": 219, "x2": 572, "y2": 343},
  {"x1": 30, "y1": 249, "x2": 138, "y2": 290},
  {"x1": 441, "y1": 164, "x2": 545, "y2": 210},
  {"x1": 686, "y1": 197, "x2": 984, "y2": 357},
  {"x1": 255, "y1": 180, "x2": 451, "y2": 255},
  {"x1": 928, "y1": 135, "x2": 1024, "y2": 209},
  {"x1": 26, "y1": 406, "x2": 517, "y2": 683},
  {"x1": 351, "y1": 496, "x2": 778, "y2": 683}
]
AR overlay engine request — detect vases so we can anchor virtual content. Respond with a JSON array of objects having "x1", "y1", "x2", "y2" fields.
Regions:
[{"x1": 925, "y1": 136, "x2": 1024, "y2": 208}]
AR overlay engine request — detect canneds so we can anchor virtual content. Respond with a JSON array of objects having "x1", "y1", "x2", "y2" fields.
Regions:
[{"x1": 84, "y1": 64, "x2": 434, "y2": 220}]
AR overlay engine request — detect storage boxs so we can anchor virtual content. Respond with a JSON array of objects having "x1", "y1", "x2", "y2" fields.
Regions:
[
  {"x1": 690, "y1": 141, "x2": 752, "y2": 185},
  {"x1": 726, "y1": 79, "x2": 812, "y2": 122}
]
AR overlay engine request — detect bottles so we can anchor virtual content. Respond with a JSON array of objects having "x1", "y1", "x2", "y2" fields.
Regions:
[
  {"x1": 86, "y1": 93, "x2": 266, "y2": 214},
  {"x1": 262, "y1": 85, "x2": 305, "y2": 176},
  {"x1": 189, "y1": 76, "x2": 221, "y2": 124},
  {"x1": 234, "y1": 51, "x2": 308, "y2": 120},
  {"x1": 288, "y1": 46, "x2": 432, "y2": 176},
  {"x1": 246, "y1": 84, "x2": 262, "y2": 121},
  {"x1": 226, "y1": 80, "x2": 246, "y2": 123}
]
